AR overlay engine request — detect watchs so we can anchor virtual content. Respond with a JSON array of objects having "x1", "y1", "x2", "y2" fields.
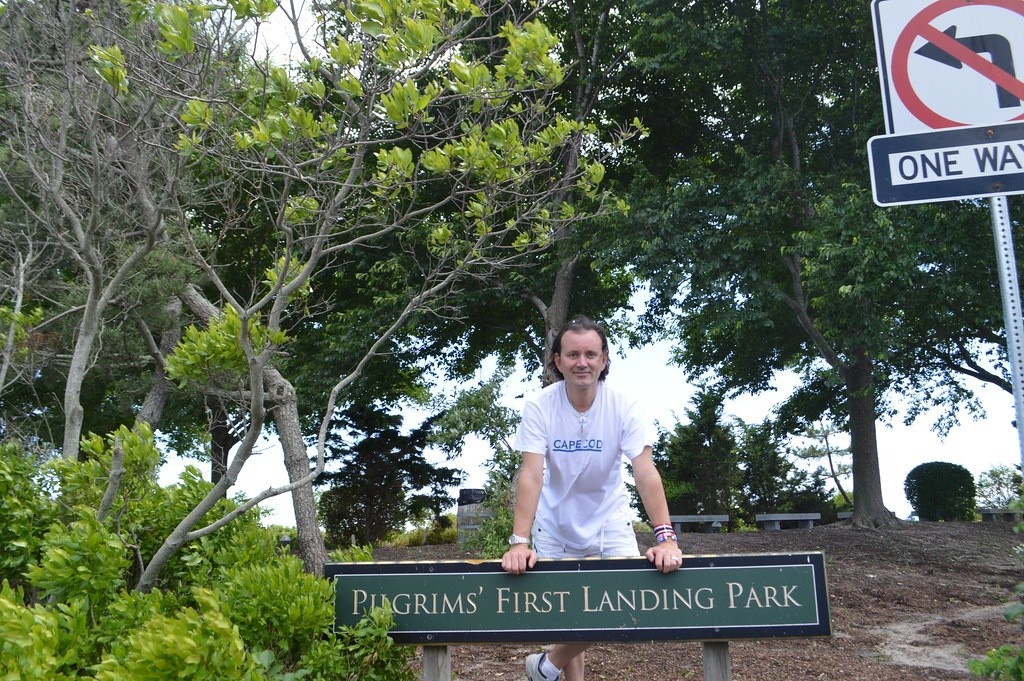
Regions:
[{"x1": 508, "y1": 535, "x2": 531, "y2": 546}]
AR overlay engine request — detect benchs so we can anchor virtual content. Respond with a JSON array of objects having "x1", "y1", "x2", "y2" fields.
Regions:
[
  {"x1": 668, "y1": 515, "x2": 729, "y2": 533},
  {"x1": 756, "y1": 513, "x2": 821, "y2": 530},
  {"x1": 837, "y1": 512, "x2": 895, "y2": 519},
  {"x1": 974, "y1": 509, "x2": 1020, "y2": 523}
]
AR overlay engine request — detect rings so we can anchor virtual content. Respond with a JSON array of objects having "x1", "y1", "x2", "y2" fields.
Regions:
[{"x1": 671, "y1": 557, "x2": 678, "y2": 562}]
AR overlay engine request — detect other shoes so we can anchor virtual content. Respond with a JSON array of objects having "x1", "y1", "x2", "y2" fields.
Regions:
[{"x1": 522, "y1": 653, "x2": 560, "y2": 681}]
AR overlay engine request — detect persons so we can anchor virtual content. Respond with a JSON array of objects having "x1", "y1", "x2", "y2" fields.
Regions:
[{"x1": 502, "y1": 317, "x2": 682, "y2": 681}]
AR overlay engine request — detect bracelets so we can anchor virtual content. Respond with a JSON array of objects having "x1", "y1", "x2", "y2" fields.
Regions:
[{"x1": 654, "y1": 523, "x2": 678, "y2": 543}]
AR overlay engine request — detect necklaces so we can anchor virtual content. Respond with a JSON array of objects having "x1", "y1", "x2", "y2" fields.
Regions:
[{"x1": 567, "y1": 387, "x2": 597, "y2": 423}]
